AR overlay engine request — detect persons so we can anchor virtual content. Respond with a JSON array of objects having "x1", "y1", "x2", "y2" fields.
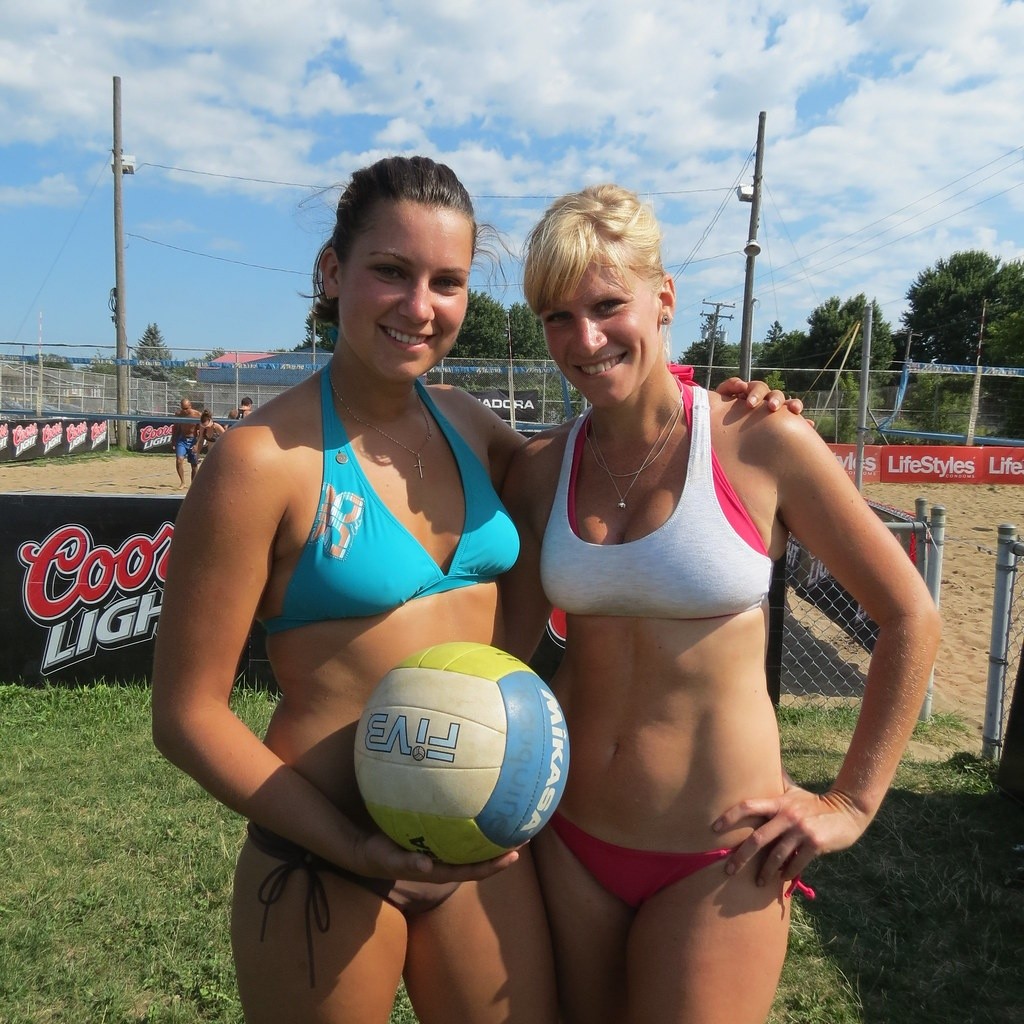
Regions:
[
  {"x1": 172, "y1": 396, "x2": 253, "y2": 489},
  {"x1": 498, "y1": 184, "x2": 943, "y2": 1024},
  {"x1": 151, "y1": 156, "x2": 815, "y2": 1024}
]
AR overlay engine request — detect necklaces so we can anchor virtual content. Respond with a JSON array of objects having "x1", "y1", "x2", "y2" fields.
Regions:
[
  {"x1": 329, "y1": 365, "x2": 432, "y2": 479},
  {"x1": 584, "y1": 377, "x2": 684, "y2": 509}
]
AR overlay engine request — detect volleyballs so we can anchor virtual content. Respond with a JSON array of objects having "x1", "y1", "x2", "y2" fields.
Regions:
[{"x1": 351, "y1": 641, "x2": 573, "y2": 866}]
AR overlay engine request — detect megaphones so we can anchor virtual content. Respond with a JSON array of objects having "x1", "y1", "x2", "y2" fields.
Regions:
[{"x1": 744, "y1": 239, "x2": 761, "y2": 256}]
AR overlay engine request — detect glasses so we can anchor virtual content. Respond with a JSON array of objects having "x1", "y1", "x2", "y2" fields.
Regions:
[{"x1": 199, "y1": 419, "x2": 213, "y2": 429}]
